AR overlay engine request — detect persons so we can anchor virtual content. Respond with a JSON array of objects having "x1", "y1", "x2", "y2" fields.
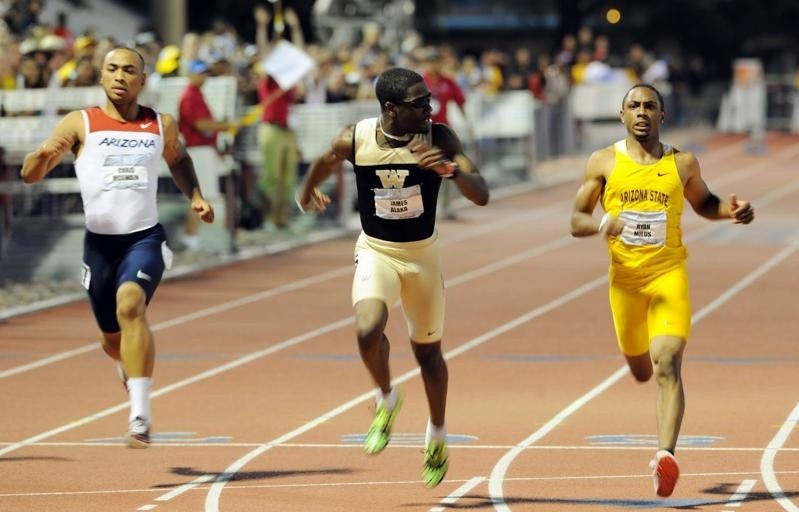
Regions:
[
  {"x1": 0, "y1": 1, "x2": 677, "y2": 250},
  {"x1": 569, "y1": 85, "x2": 755, "y2": 496},
  {"x1": 294, "y1": 69, "x2": 491, "y2": 488},
  {"x1": 19, "y1": 45, "x2": 215, "y2": 447}
]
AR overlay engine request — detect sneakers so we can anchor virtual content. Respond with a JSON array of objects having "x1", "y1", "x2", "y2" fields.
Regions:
[
  {"x1": 126, "y1": 418, "x2": 150, "y2": 449},
  {"x1": 421, "y1": 441, "x2": 447, "y2": 491},
  {"x1": 362, "y1": 388, "x2": 404, "y2": 455},
  {"x1": 650, "y1": 451, "x2": 679, "y2": 497}
]
